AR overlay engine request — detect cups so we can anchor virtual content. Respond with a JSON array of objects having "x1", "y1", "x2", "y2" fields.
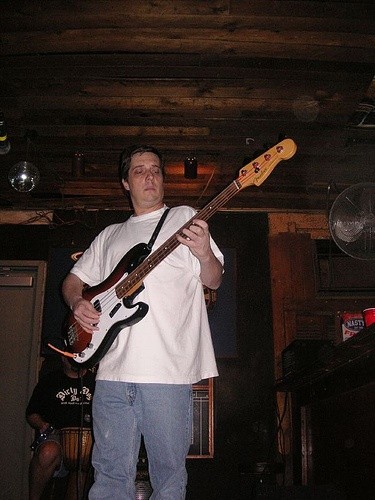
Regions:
[{"x1": 363, "y1": 308, "x2": 375, "y2": 327}]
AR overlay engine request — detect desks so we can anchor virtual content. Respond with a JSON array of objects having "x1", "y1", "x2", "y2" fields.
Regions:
[{"x1": 274, "y1": 322, "x2": 375, "y2": 500}]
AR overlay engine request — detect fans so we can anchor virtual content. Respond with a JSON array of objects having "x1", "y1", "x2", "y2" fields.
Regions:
[{"x1": 328, "y1": 182, "x2": 375, "y2": 261}]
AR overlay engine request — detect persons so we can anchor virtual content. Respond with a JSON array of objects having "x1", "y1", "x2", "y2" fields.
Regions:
[
  {"x1": 25, "y1": 354, "x2": 96, "y2": 500},
  {"x1": 63, "y1": 147, "x2": 226, "y2": 500}
]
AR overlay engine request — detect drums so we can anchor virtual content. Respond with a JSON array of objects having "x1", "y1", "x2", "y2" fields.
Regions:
[{"x1": 60, "y1": 426, "x2": 98, "y2": 500}]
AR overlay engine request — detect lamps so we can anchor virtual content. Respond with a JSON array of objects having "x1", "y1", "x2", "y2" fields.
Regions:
[
  {"x1": 8, "y1": 136, "x2": 40, "y2": 193},
  {"x1": 185, "y1": 155, "x2": 198, "y2": 179},
  {"x1": 73, "y1": 153, "x2": 85, "y2": 180}
]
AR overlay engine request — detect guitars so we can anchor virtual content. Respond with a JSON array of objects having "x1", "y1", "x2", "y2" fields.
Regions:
[{"x1": 63, "y1": 139, "x2": 295, "y2": 378}]
